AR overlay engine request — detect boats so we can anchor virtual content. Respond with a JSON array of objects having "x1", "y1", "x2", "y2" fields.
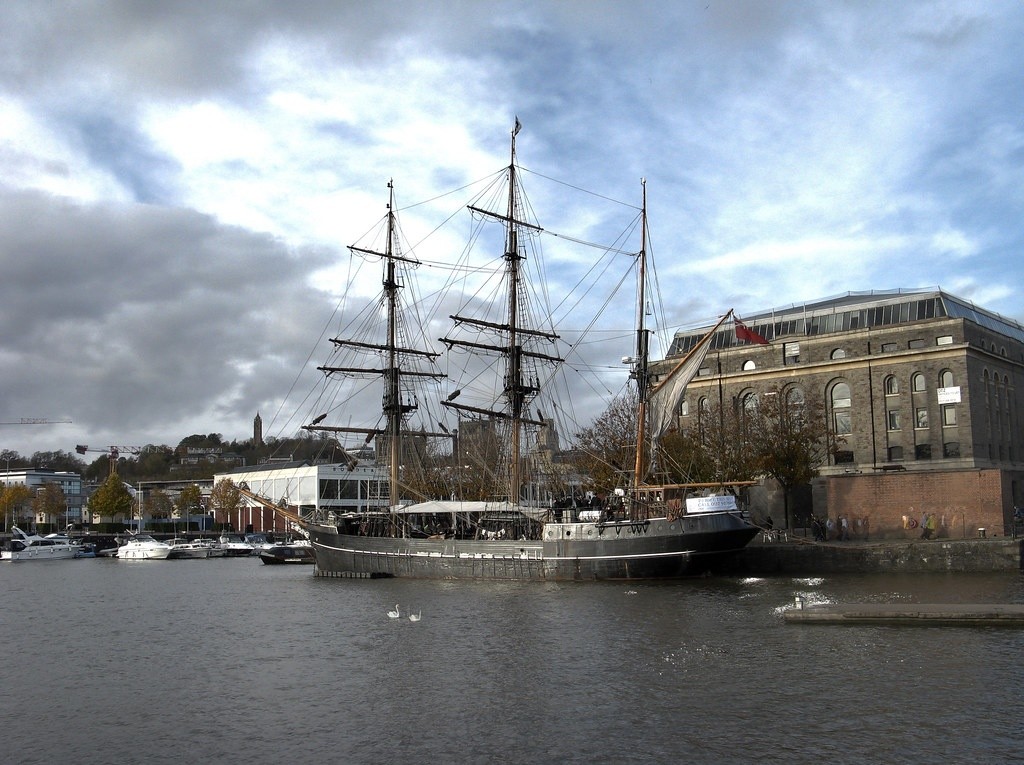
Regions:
[{"x1": 0, "y1": 524, "x2": 316, "y2": 566}]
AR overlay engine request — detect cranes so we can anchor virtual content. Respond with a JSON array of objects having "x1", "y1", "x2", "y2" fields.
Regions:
[{"x1": 73, "y1": 443, "x2": 165, "y2": 478}]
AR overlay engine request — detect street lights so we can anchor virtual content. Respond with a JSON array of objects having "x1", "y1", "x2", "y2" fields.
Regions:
[{"x1": 2, "y1": 456, "x2": 20, "y2": 532}]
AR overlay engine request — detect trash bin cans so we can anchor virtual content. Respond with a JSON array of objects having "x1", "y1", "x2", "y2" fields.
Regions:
[{"x1": 247, "y1": 524, "x2": 254, "y2": 534}]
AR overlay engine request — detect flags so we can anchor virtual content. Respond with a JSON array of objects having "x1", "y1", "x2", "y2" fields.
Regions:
[{"x1": 733, "y1": 315, "x2": 770, "y2": 344}]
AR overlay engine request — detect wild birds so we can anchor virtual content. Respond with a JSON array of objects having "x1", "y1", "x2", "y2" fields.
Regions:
[
  {"x1": 408, "y1": 610, "x2": 421, "y2": 622},
  {"x1": 386, "y1": 604, "x2": 400, "y2": 618}
]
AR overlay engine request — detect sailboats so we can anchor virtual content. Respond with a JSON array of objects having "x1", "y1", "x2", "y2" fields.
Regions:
[{"x1": 233, "y1": 116, "x2": 766, "y2": 585}]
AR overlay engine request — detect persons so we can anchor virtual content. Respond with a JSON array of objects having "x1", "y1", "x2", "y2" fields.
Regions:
[
  {"x1": 766, "y1": 516, "x2": 773, "y2": 529},
  {"x1": 920, "y1": 511, "x2": 937, "y2": 541},
  {"x1": 553, "y1": 492, "x2": 601, "y2": 523},
  {"x1": 794, "y1": 513, "x2": 870, "y2": 542},
  {"x1": 1013, "y1": 506, "x2": 1021, "y2": 522}
]
